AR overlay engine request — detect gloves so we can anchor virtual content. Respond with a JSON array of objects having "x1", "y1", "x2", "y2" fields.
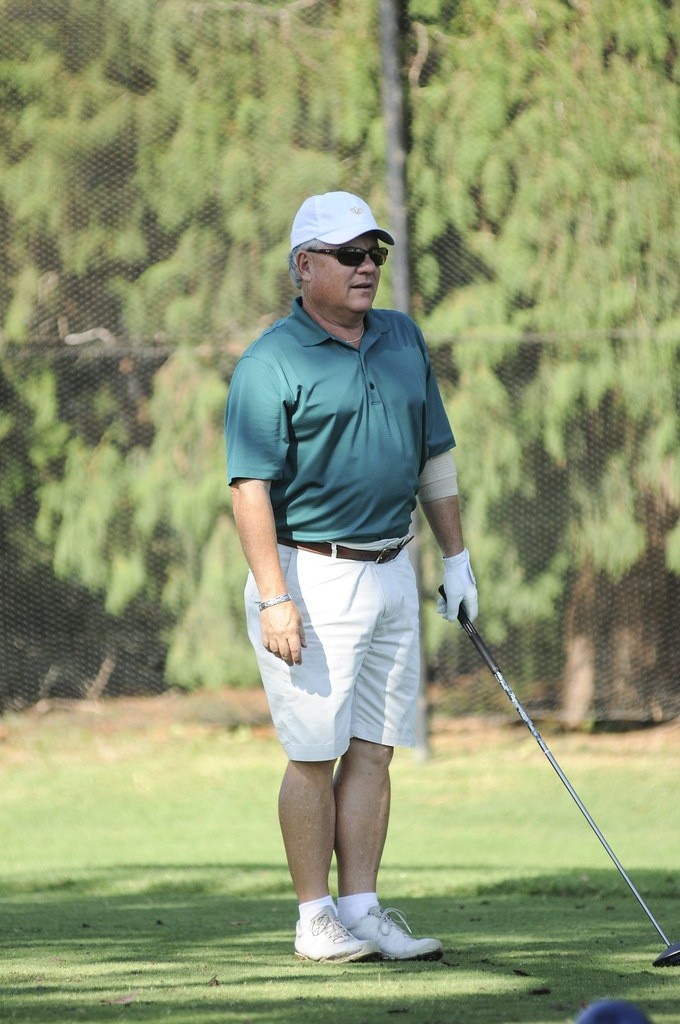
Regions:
[{"x1": 436, "y1": 548, "x2": 479, "y2": 624}]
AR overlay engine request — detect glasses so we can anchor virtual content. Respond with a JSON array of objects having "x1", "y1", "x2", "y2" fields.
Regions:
[{"x1": 306, "y1": 246, "x2": 388, "y2": 270}]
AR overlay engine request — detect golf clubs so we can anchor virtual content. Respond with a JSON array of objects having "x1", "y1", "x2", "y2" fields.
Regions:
[{"x1": 438, "y1": 582, "x2": 679, "y2": 968}]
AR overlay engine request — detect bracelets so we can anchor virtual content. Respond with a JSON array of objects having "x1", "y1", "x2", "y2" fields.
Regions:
[{"x1": 258, "y1": 594, "x2": 293, "y2": 613}]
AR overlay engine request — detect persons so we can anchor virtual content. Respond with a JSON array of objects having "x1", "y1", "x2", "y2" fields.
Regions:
[{"x1": 224, "y1": 190, "x2": 479, "y2": 962}]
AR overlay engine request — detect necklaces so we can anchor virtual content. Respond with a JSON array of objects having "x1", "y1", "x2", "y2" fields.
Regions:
[{"x1": 342, "y1": 325, "x2": 367, "y2": 347}]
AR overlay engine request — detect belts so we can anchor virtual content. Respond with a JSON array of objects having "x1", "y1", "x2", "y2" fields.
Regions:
[{"x1": 276, "y1": 534, "x2": 416, "y2": 565}]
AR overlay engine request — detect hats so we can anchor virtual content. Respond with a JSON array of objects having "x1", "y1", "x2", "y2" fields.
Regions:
[{"x1": 291, "y1": 191, "x2": 395, "y2": 254}]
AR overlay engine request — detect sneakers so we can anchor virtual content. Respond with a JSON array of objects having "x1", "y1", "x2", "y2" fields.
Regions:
[
  {"x1": 293, "y1": 904, "x2": 383, "y2": 964},
  {"x1": 348, "y1": 901, "x2": 443, "y2": 960}
]
